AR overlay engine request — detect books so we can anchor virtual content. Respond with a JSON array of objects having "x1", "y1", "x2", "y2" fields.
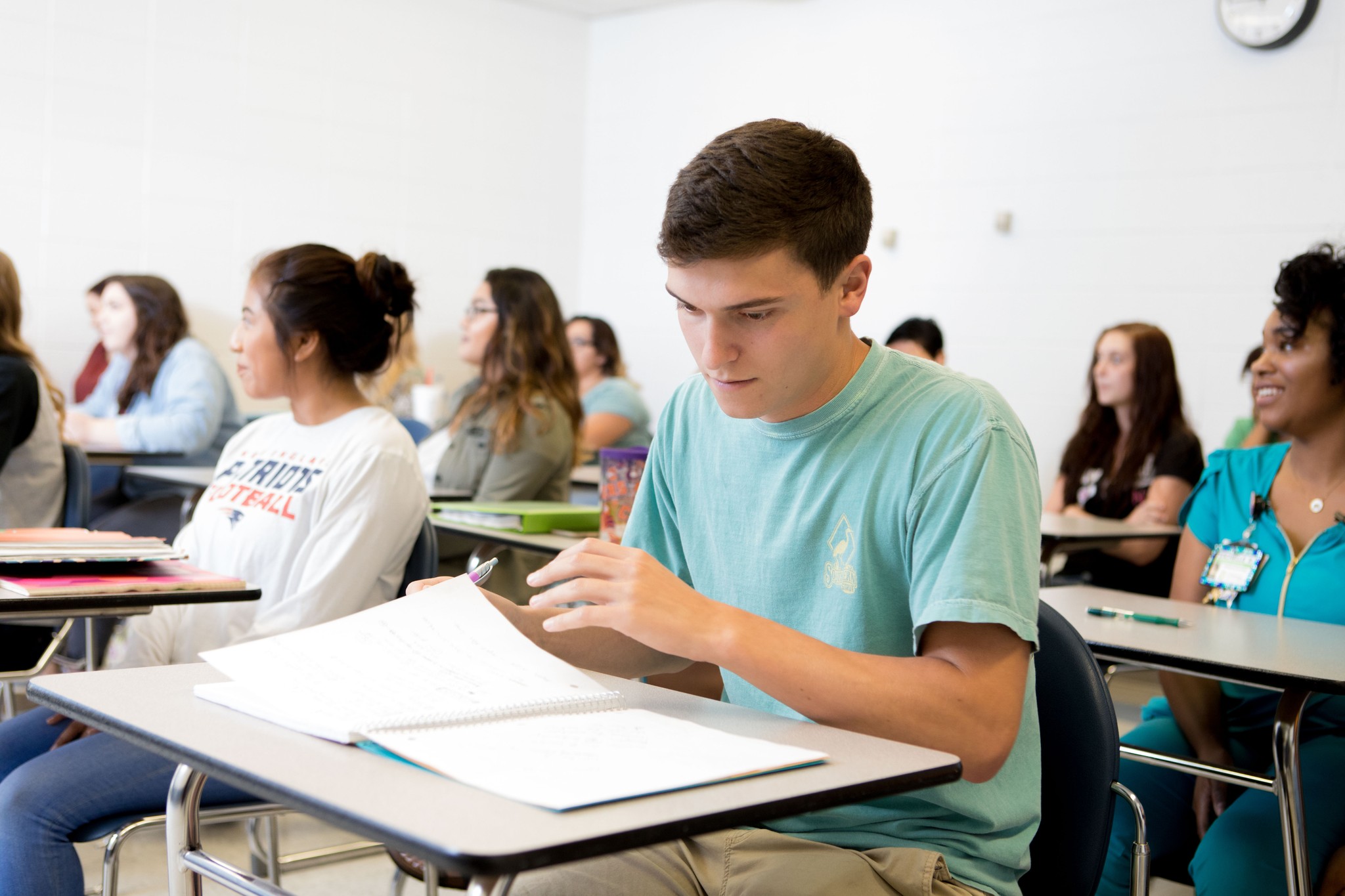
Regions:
[{"x1": 196, "y1": 575, "x2": 831, "y2": 819}]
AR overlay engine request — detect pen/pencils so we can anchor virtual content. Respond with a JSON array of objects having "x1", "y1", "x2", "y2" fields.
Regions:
[
  {"x1": 468, "y1": 556, "x2": 499, "y2": 586},
  {"x1": 1087, "y1": 607, "x2": 1180, "y2": 627}
]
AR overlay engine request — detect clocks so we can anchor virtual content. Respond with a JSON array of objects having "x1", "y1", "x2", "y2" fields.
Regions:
[{"x1": 1215, "y1": 0, "x2": 1319, "y2": 50}]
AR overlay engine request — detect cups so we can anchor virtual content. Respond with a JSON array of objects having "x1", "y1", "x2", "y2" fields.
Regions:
[
  {"x1": 594, "y1": 444, "x2": 651, "y2": 544},
  {"x1": 413, "y1": 385, "x2": 446, "y2": 427}
]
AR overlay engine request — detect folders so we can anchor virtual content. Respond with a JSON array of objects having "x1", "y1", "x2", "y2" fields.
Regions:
[{"x1": 428, "y1": 502, "x2": 601, "y2": 534}]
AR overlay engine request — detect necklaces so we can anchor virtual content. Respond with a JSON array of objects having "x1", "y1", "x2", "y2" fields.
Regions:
[{"x1": 1290, "y1": 464, "x2": 1344, "y2": 512}]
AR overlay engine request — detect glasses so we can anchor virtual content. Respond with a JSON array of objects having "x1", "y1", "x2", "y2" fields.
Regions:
[{"x1": 466, "y1": 306, "x2": 503, "y2": 322}]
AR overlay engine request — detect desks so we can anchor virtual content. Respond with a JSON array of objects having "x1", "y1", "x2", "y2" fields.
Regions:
[{"x1": 0, "y1": 448, "x2": 1345, "y2": 896}]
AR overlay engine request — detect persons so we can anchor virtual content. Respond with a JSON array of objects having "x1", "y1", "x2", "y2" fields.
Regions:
[
  {"x1": 0, "y1": 237, "x2": 440, "y2": 895},
  {"x1": 562, "y1": 315, "x2": 654, "y2": 506},
  {"x1": 0, "y1": 248, "x2": 69, "y2": 675},
  {"x1": 1099, "y1": 244, "x2": 1345, "y2": 895},
  {"x1": 1042, "y1": 325, "x2": 1203, "y2": 597},
  {"x1": 884, "y1": 318, "x2": 946, "y2": 367},
  {"x1": 398, "y1": 121, "x2": 1045, "y2": 896},
  {"x1": 1225, "y1": 342, "x2": 1280, "y2": 446},
  {"x1": 413, "y1": 267, "x2": 585, "y2": 605},
  {"x1": 43, "y1": 274, "x2": 243, "y2": 688}
]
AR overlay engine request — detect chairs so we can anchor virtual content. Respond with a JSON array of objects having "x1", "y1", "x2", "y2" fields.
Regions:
[
  {"x1": 77, "y1": 511, "x2": 438, "y2": 896},
  {"x1": 1020, "y1": 600, "x2": 1150, "y2": 896},
  {"x1": 0, "y1": 484, "x2": 196, "y2": 717},
  {"x1": 54, "y1": 445, "x2": 94, "y2": 531}
]
